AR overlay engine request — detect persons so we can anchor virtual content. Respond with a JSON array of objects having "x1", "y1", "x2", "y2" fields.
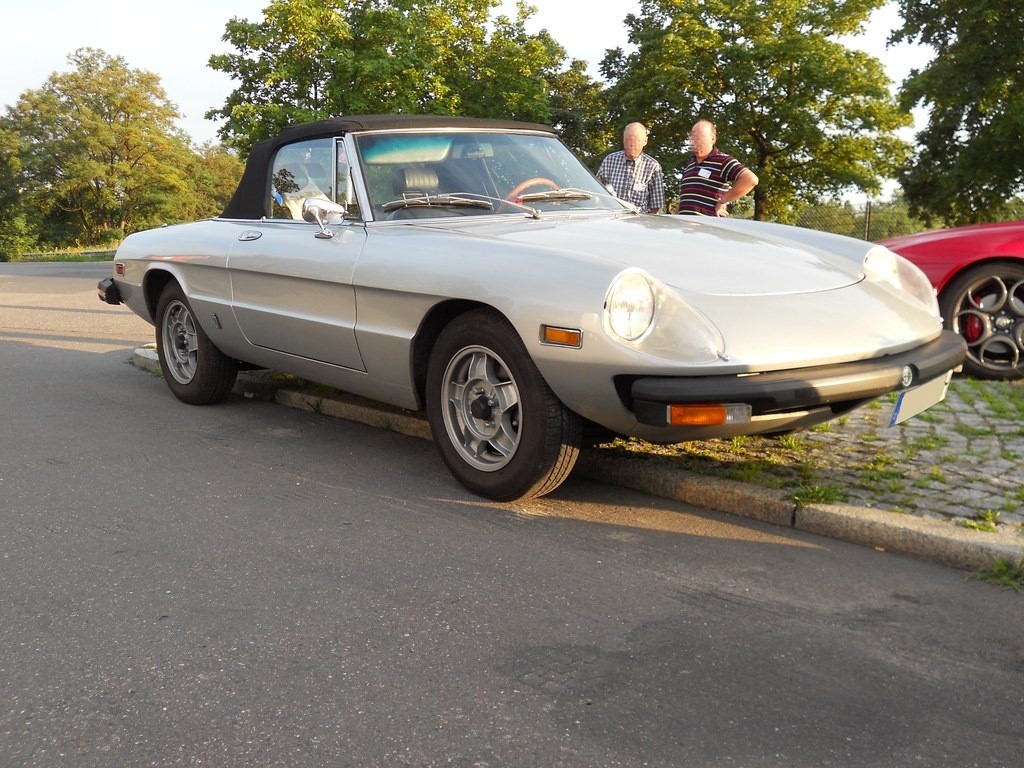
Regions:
[
  {"x1": 596, "y1": 122, "x2": 665, "y2": 215},
  {"x1": 675, "y1": 121, "x2": 760, "y2": 218}
]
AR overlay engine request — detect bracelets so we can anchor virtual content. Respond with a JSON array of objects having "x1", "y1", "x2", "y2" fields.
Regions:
[{"x1": 718, "y1": 198, "x2": 728, "y2": 205}]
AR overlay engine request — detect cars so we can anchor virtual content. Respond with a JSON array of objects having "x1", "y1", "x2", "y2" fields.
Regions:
[
  {"x1": 872, "y1": 222, "x2": 1024, "y2": 381},
  {"x1": 97, "y1": 112, "x2": 969, "y2": 504}
]
